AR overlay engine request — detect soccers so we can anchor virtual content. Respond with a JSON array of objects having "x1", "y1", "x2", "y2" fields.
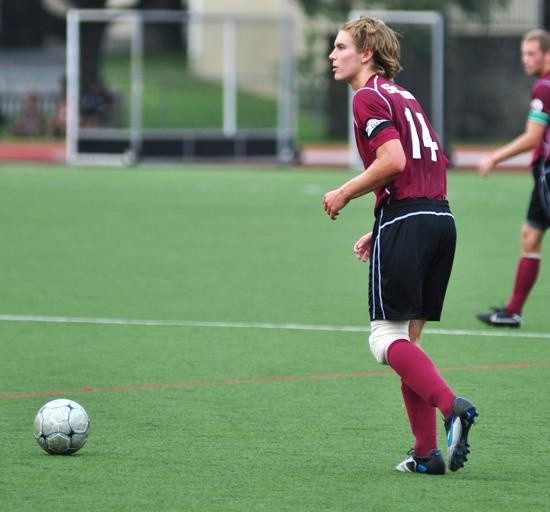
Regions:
[{"x1": 33, "y1": 400, "x2": 91, "y2": 455}]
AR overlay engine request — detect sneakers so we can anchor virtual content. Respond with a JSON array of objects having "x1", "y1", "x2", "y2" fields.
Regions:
[
  {"x1": 476, "y1": 307, "x2": 523, "y2": 327},
  {"x1": 444, "y1": 397, "x2": 479, "y2": 472},
  {"x1": 396, "y1": 448, "x2": 446, "y2": 475}
]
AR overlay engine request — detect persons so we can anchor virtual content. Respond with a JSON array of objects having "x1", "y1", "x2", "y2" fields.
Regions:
[
  {"x1": 320, "y1": 16, "x2": 482, "y2": 476},
  {"x1": 476, "y1": 27, "x2": 550, "y2": 328}
]
